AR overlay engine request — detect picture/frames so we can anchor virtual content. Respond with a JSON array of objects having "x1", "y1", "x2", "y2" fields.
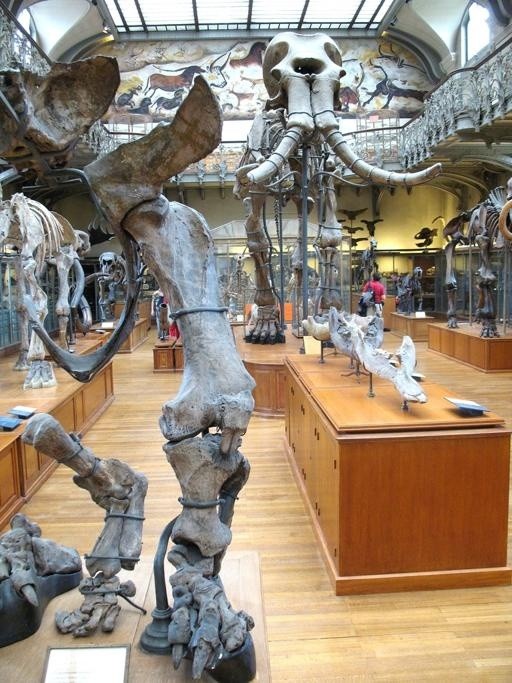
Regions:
[{"x1": 45, "y1": 643, "x2": 129, "y2": 683}]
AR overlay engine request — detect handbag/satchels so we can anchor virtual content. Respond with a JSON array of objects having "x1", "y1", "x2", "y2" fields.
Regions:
[{"x1": 362, "y1": 280, "x2": 375, "y2": 307}]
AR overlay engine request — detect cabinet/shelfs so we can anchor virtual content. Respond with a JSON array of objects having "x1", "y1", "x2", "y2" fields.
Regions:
[{"x1": 281, "y1": 352, "x2": 512, "y2": 597}]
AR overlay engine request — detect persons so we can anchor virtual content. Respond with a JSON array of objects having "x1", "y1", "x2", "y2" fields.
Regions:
[
  {"x1": 248, "y1": 299, "x2": 259, "y2": 327},
  {"x1": 361, "y1": 271, "x2": 387, "y2": 319},
  {"x1": 151, "y1": 285, "x2": 180, "y2": 339}
]
